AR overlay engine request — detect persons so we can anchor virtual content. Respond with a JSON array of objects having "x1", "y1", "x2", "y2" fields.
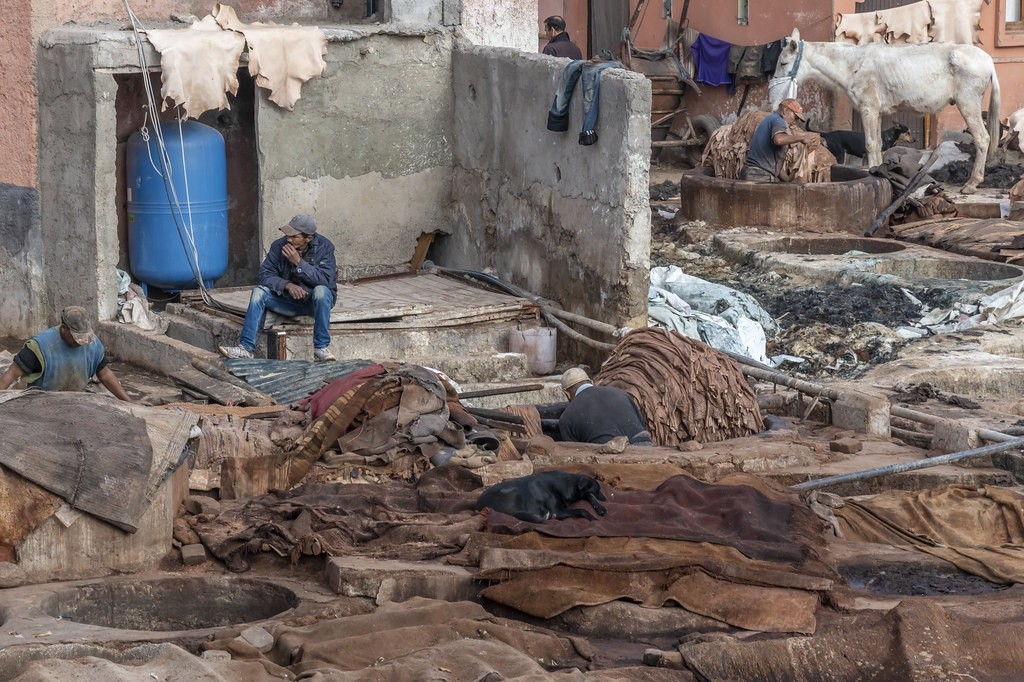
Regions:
[
  {"x1": 219, "y1": 215, "x2": 338, "y2": 362},
  {"x1": 557, "y1": 368, "x2": 653, "y2": 445},
  {"x1": 0, "y1": 306, "x2": 153, "y2": 406},
  {"x1": 542, "y1": 15, "x2": 582, "y2": 60},
  {"x1": 740, "y1": 98, "x2": 817, "y2": 183}
]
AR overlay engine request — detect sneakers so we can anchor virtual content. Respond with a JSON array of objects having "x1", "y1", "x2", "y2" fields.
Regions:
[
  {"x1": 314, "y1": 349, "x2": 336, "y2": 361},
  {"x1": 219, "y1": 344, "x2": 254, "y2": 359}
]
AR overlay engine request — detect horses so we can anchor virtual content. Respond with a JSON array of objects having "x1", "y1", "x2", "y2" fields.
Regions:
[{"x1": 767, "y1": 28, "x2": 1004, "y2": 194}]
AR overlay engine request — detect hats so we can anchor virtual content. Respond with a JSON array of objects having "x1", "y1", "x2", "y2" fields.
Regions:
[
  {"x1": 279, "y1": 214, "x2": 317, "y2": 236},
  {"x1": 62, "y1": 305, "x2": 96, "y2": 345},
  {"x1": 561, "y1": 367, "x2": 591, "y2": 390},
  {"x1": 781, "y1": 97, "x2": 806, "y2": 122}
]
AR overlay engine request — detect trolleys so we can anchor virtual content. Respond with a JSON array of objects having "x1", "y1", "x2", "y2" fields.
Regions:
[{"x1": 618, "y1": 0, "x2": 722, "y2": 169}]
